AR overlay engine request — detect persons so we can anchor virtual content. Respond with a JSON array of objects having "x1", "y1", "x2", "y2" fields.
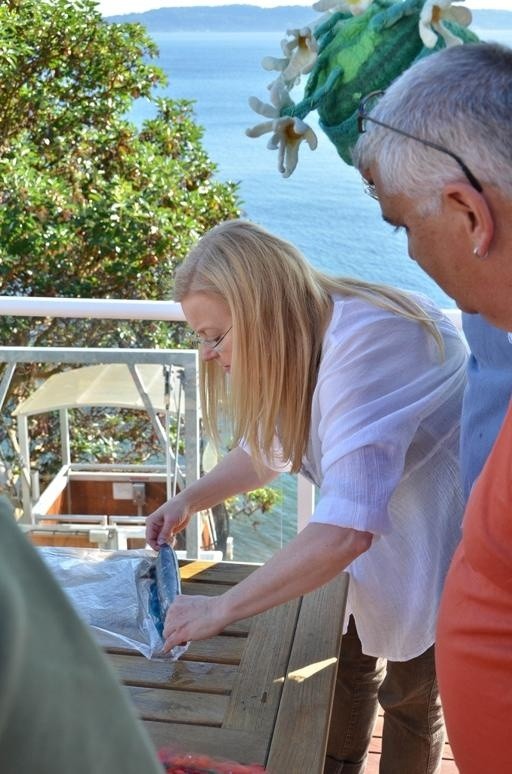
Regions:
[
  {"x1": 314, "y1": 17, "x2": 509, "y2": 503},
  {"x1": 350, "y1": 38, "x2": 512, "y2": 773},
  {"x1": 144, "y1": 217, "x2": 469, "y2": 773}
]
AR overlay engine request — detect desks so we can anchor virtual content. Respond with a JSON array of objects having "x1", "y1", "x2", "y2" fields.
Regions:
[{"x1": 35, "y1": 543, "x2": 350, "y2": 773}]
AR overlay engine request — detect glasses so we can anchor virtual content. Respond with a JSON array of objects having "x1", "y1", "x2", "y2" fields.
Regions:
[
  {"x1": 189, "y1": 326, "x2": 233, "y2": 352},
  {"x1": 356, "y1": 89, "x2": 484, "y2": 196}
]
